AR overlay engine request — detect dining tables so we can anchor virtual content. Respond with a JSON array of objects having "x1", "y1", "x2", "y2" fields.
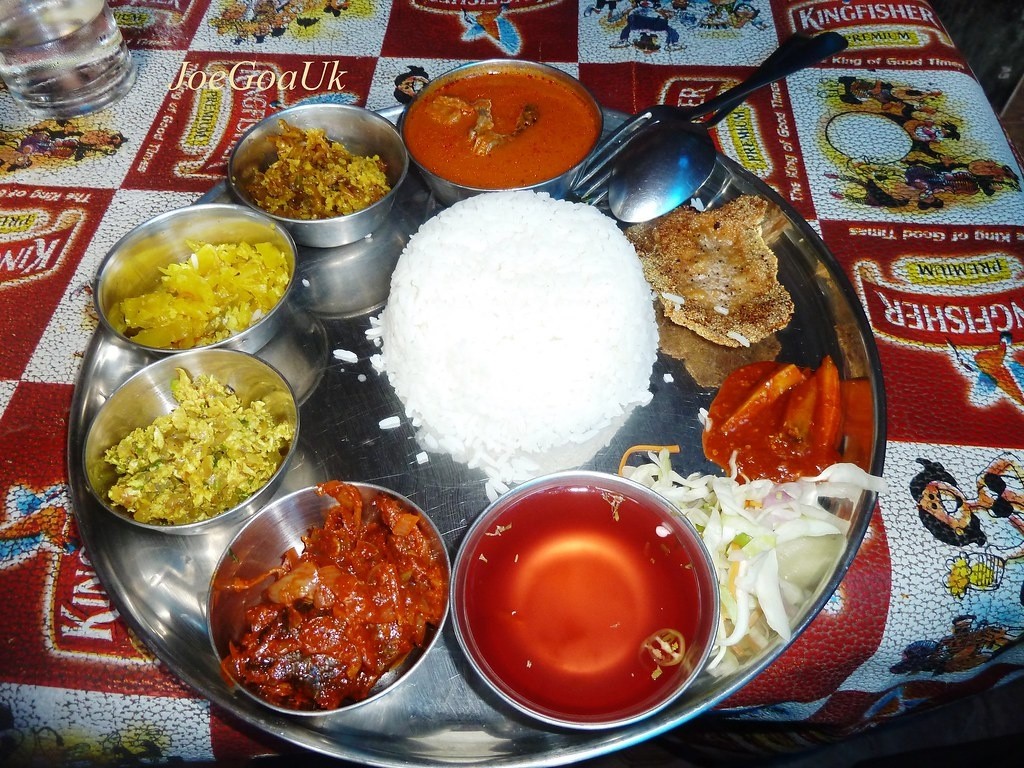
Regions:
[{"x1": 0, "y1": 0, "x2": 1024, "y2": 768}]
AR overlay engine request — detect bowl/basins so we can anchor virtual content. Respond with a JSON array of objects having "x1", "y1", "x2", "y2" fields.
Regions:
[
  {"x1": 96, "y1": 203, "x2": 300, "y2": 362},
  {"x1": 84, "y1": 348, "x2": 301, "y2": 537},
  {"x1": 206, "y1": 480, "x2": 452, "y2": 717},
  {"x1": 451, "y1": 469, "x2": 719, "y2": 731},
  {"x1": 228, "y1": 103, "x2": 411, "y2": 248},
  {"x1": 400, "y1": 58, "x2": 604, "y2": 209}
]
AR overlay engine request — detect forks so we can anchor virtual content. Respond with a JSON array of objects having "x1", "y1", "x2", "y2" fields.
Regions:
[{"x1": 573, "y1": 31, "x2": 848, "y2": 206}]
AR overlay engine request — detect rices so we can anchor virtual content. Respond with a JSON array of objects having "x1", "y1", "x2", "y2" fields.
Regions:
[{"x1": 333, "y1": 189, "x2": 660, "y2": 498}]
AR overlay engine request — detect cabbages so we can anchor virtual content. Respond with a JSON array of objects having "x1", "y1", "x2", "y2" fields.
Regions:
[{"x1": 623, "y1": 451, "x2": 891, "y2": 668}]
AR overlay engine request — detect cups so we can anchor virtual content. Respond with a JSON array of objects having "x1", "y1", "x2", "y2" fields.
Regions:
[{"x1": 0, "y1": 0, "x2": 138, "y2": 120}]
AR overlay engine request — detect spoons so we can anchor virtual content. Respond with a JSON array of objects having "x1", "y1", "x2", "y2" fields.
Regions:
[{"x1": 608, "y1": 29, "x2": 816, "y2": 222}]
